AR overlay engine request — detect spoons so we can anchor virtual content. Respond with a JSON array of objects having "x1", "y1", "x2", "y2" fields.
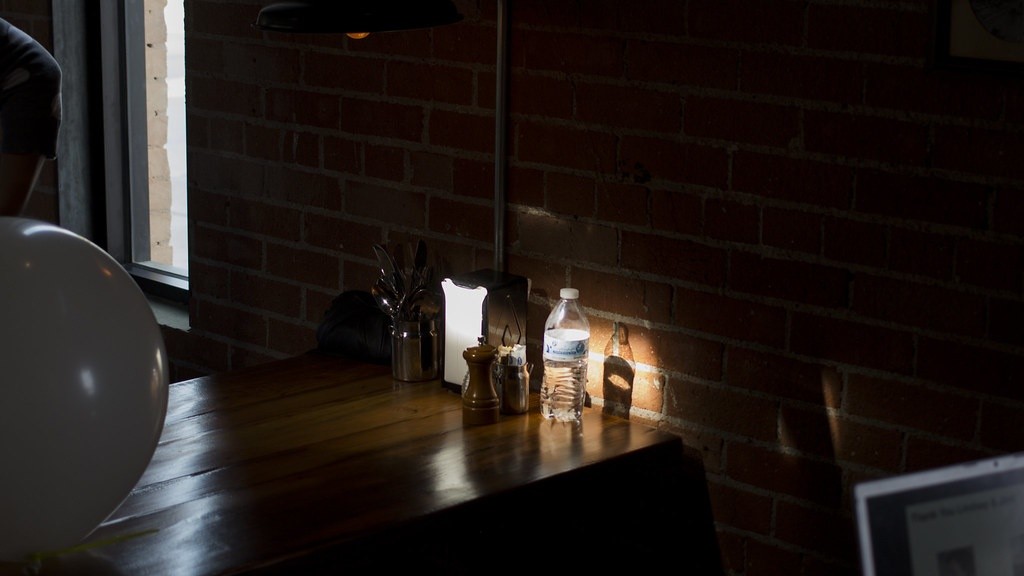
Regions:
[{"x1": 372, "y1": 278, "x2": 398, "y2": 320}]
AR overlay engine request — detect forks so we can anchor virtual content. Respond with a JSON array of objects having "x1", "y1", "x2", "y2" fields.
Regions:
[{"x1": 409, "y1": 256, "x2": 445, "y2": 307}]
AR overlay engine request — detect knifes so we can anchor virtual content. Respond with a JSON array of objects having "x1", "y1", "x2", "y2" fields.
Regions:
[{"x1": 371, "y1": 238, "x2": 427, "y2": 319}]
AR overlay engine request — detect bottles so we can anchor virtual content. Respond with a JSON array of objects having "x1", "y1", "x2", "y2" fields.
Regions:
[{"x1": 540, "y1": 288, "x2": 590, "y2": 422}]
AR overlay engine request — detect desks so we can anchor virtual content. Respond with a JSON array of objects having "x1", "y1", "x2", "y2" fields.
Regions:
[{"x1": 0, "y1": 352, "x2": 683, "y2": 576}]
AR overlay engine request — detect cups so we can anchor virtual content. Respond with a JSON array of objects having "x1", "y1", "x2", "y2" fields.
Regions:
[
  {"x1": 390, "y1": 318, "x2": 439, "y2": 382},
  {"x1": 498, "y1": 364, "x2": 529, "y2": 414}
]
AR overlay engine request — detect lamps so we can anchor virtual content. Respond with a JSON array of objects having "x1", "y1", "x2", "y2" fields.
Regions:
[{"x1": 251, "y1": 0, "x2": 529, "y2": 396}]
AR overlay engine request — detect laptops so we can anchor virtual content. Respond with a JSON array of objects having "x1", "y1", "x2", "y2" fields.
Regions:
[{"x1": 858, "y1": 453, "x2": 1024, "y2": 576}]
